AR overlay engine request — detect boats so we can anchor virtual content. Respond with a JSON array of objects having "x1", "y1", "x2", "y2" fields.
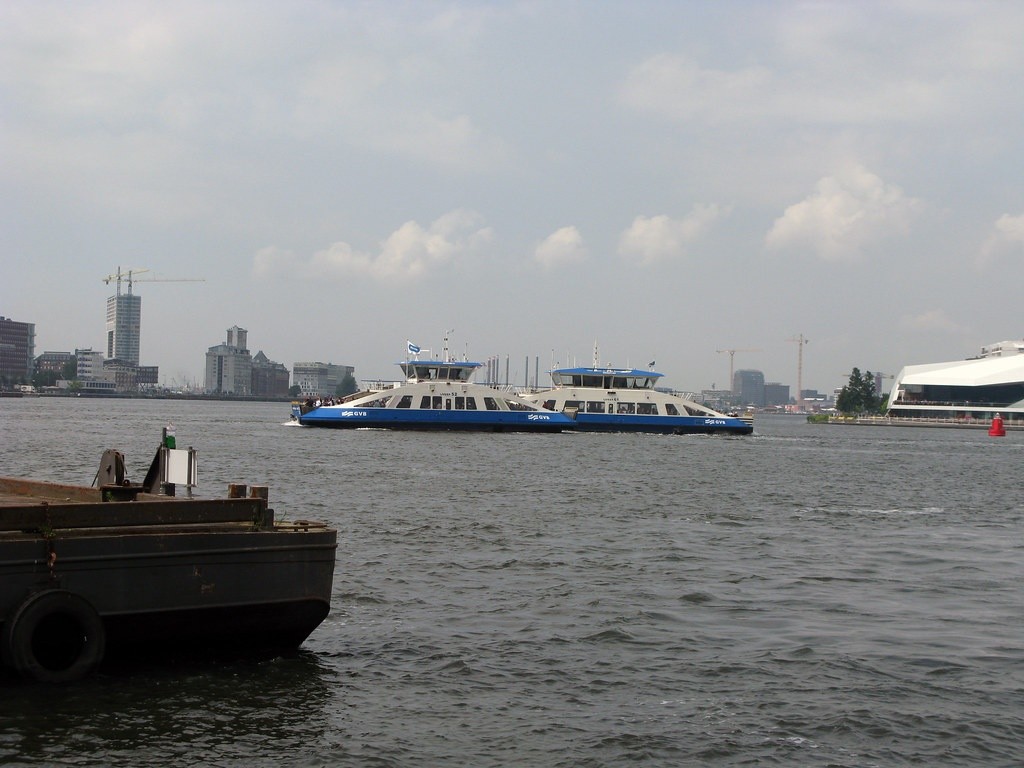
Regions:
[
  {"x1": 290, "y1": 401, "x2": 318, "y2": 421},
  {"x1": 520, "y1": 338, "x2": 754, "y2": 435},
  {"x1": 296, "y1": 330, "x2": 581, "y2": 434}
]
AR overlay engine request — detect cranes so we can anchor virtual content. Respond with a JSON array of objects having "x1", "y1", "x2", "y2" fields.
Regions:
[
  {"x1": 784, "y1": 332, "x2": 809, "y2": 404},
  {"x1": 842, "y1": 372, "x2": 894, "y2": 393},
  {"x1": 100, "y1": 264, "x2": 207, "y2": 294},
  {"x1": 714, "y1": 349, "x2": 736, "y2": 397}
]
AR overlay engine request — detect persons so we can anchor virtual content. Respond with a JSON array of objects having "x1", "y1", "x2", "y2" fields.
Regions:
[
  {"x1": 302, "y1": 395, "x2": 343, "y2": 408},
  {"x1": 609, "y1": 400, "x2": 739, "y2": 416}
]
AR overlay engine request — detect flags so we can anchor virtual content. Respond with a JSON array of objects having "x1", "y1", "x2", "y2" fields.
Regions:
[{"x1": 407, "y1": 340, "x2": 420, "y2": 355}]
